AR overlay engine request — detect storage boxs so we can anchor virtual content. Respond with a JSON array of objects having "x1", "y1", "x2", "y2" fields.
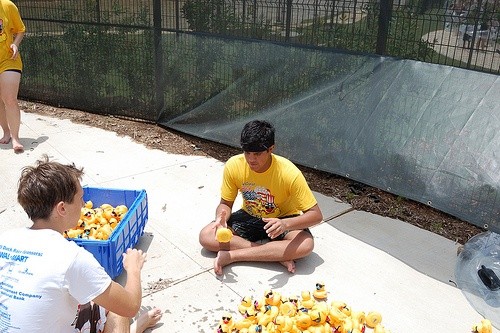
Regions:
[{"x1": 63, "y1": 185, "x2": 148, "y2": 281}]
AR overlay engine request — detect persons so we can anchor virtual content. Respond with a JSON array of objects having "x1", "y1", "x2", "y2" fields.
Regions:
[
  {"x1": 0, "y1": 0, "x2": 26, "y2": 151},
  {"x1": 199, "y1": 119, "x2": 323, "y2": 274},
  {"x1": 0, "y1": 154, "x2": 162, "y2": 333}
]
